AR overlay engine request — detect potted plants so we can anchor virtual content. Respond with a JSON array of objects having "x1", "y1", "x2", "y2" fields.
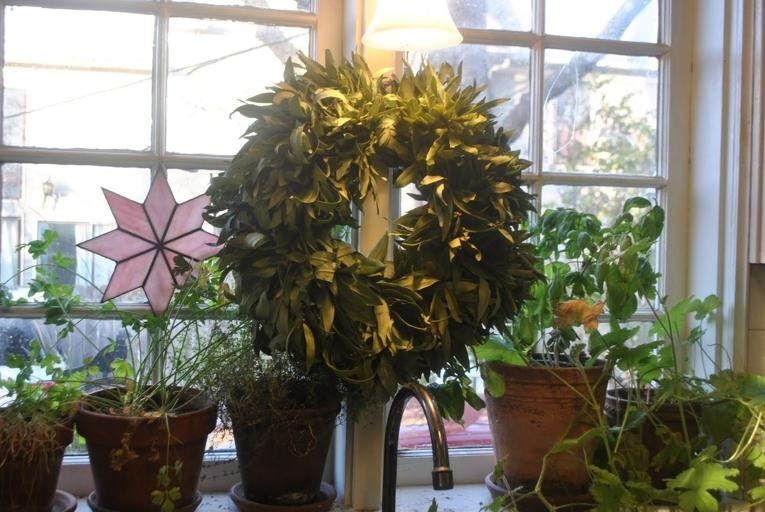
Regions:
[
  {"x1": 76, "y1": 253, "x2": 248, "y2": 512},
  {"x1": 200, "y1": 318, "x2": 361, "y2": 511},
  {"x1": 473, "y1": 195, "x2": 765, "y2": 512},
  {"x1": 0, "y1": 228, "x2": 104, "y2": 512}
]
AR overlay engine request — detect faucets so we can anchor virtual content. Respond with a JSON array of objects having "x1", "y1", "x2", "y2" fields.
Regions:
[{"x1": 381, "y1": 380, "x2": 455, "y2": 511}]
